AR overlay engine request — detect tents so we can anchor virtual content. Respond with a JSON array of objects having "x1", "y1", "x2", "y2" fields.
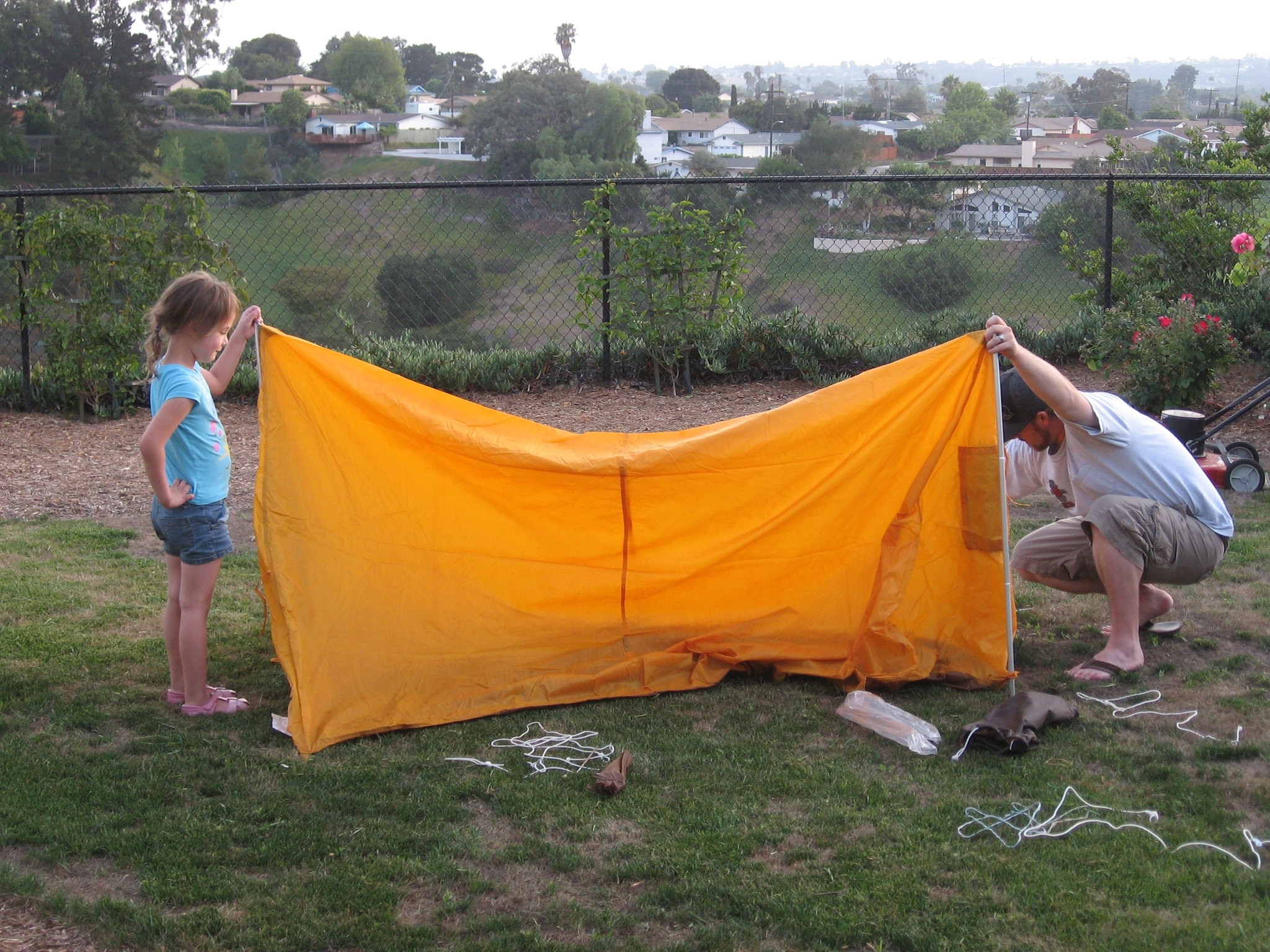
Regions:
[{"x1": 246, "y1": 306, "x2": 1016, "y2": 764}]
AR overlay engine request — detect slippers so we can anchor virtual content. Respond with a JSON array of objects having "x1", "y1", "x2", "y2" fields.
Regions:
[
  {"x1": 1063, "y1": 655, "x2": 1131, "y2": 682},
  {"x1": 1100, "y1": 619, "x2": 1184, "y2": 636}
]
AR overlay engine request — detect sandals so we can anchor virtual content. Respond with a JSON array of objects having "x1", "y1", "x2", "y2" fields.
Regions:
[
  {"x1": 166, "y1": 681, "x2": 238, "y2": 706},
  {"x1": 180, "y1": 692, "x2": 251, "y2": 718}
]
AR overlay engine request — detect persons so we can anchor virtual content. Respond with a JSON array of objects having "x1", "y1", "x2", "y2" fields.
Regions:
[
  {"x1": 138, "y1": 269, "x2": 262, "y2": 717},
  {"x1": 986, "y1": 314, "x2": 1234, "y2": 687}
]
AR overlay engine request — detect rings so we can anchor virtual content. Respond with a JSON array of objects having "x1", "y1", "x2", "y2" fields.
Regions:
[{"x1": 998, "y1": 335, "x2": 1004, "y2": 342}]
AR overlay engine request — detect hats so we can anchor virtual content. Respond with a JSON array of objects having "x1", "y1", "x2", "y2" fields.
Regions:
[{"x1": 999, "y1": 365, "x2": 1045, "y2": 442}]
